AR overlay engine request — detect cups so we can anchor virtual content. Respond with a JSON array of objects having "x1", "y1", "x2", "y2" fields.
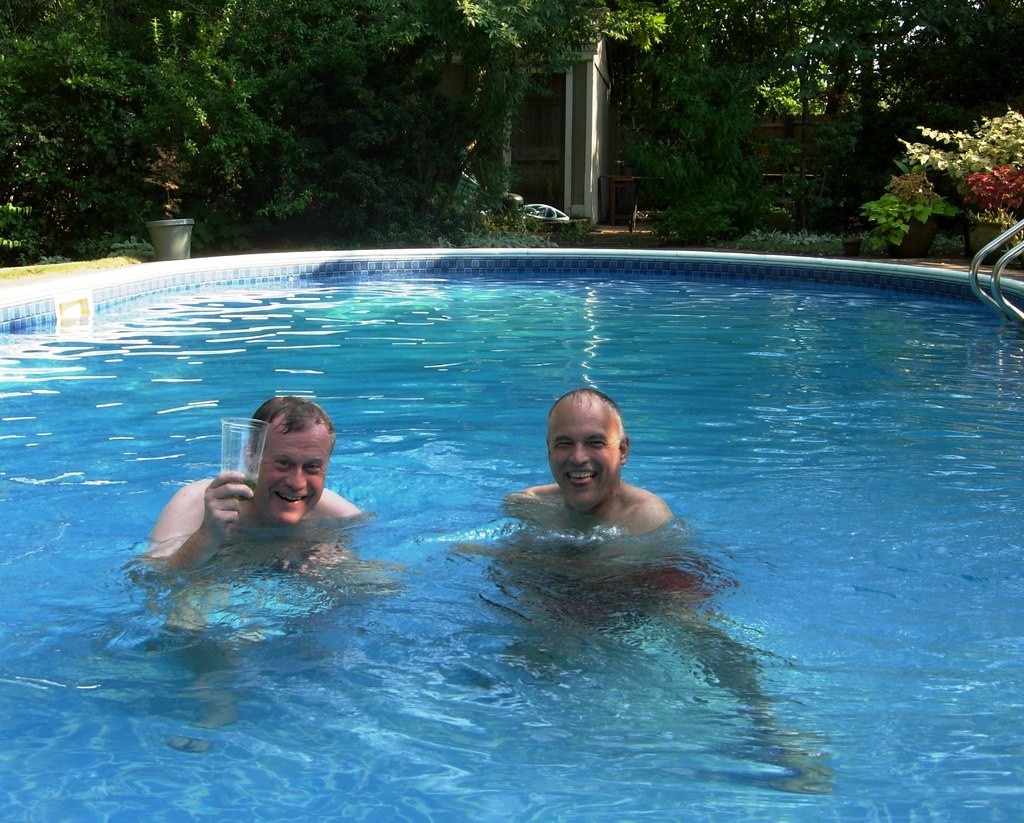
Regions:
[{"x1": 220, "y1": 417, "x2": 271, "y2": 502}]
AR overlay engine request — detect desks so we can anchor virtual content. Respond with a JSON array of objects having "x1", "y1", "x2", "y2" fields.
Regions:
[{"x1": 609, "y1": 175, "x2": 665, "y2": 226}]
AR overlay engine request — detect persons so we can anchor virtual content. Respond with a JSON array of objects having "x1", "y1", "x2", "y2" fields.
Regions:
[
  {"x1": 125, "y1": 393, "x2": 370, "y2": 568},
  {"x1": 513, "y1": 386, "x2": 671, "y2": 535}
]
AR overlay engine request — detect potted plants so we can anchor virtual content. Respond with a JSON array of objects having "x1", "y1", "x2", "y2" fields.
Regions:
[
  {"x1": 859, "y1": 171, "x2": 965, "y2": 259},
  {"x1": 962, "y1": 164, "x2": 1024, "y2": 265},
  {"x1": 842, "y1": 216, "x2": 863, "y2": 257},
  {"x1": 143, "y1": 145, "x2": 195, "y2": 261}
]
